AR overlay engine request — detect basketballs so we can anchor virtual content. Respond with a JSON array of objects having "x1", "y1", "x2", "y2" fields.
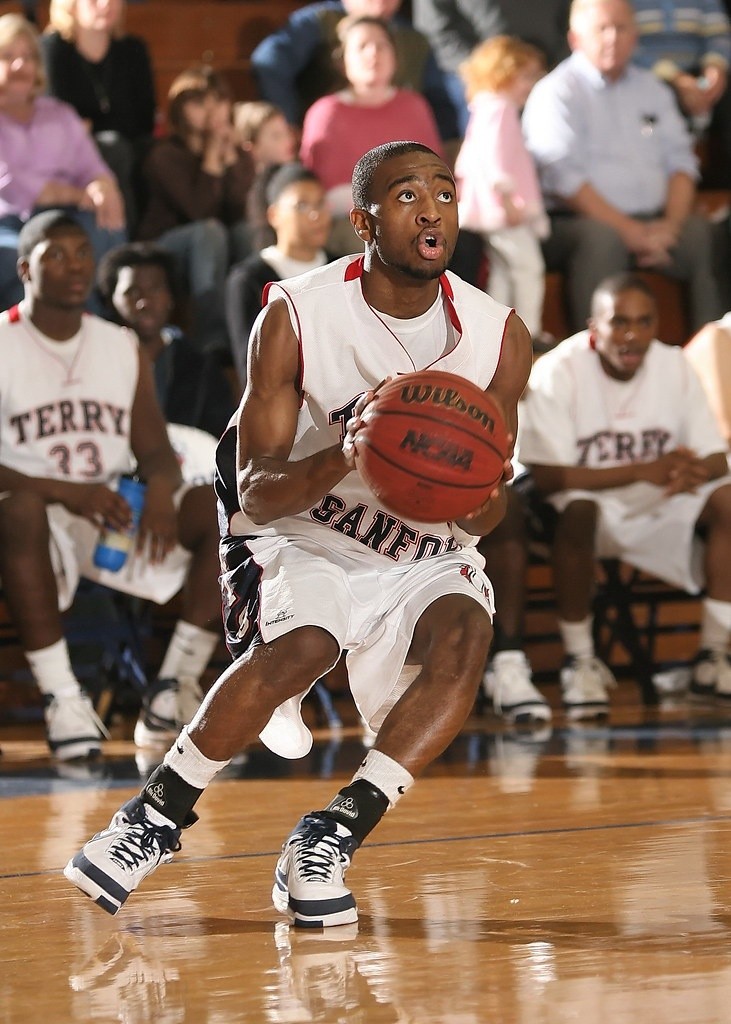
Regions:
[{"x1": 356, "y1": 369, "x2": 510, "y2": 524}]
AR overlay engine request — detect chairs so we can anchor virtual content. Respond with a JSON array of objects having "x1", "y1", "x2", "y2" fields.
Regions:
[
  {"x1": 1, "y1": 576, "x2": 171, "y2": 726},
  {"x1": 520, "y1": 548, "x2": 706, "y2": 707}
]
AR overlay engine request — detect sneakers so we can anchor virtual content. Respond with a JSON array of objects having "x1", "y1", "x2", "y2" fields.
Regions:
[
  {"x1": 64, "y1": 767, "x2": 199, "y2": 918},
  {"x1": 272, "y1": 813, "x2": 358, "y2": 928},
  {"x1": 688, "y1": 649, "x2": 731, "y2": 698},
  {"x1": 133, "y1": 676, "x2": 206, "y2": 749},
  {"x1": 560, "y1": 652, "x2": 617, "y2": 707},
  {"x1": 481, "y1": 650, "x2": 545, "y2": 715},
  {"x1": 44, "y1": 679, "x2": 110, "y2": 760}
]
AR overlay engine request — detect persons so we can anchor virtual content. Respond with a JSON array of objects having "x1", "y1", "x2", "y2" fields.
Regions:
[
  {"x1": 0, "y1": 0, "x2": 731, "y2": 765},
  {"x1": 61, "y1": 141, "x2": 535, "y2": 928}
]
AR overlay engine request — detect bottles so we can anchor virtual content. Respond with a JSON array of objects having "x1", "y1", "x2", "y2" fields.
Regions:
[{"x1": 92, "y1": 475, "x2": 148, "y2": 572}]
